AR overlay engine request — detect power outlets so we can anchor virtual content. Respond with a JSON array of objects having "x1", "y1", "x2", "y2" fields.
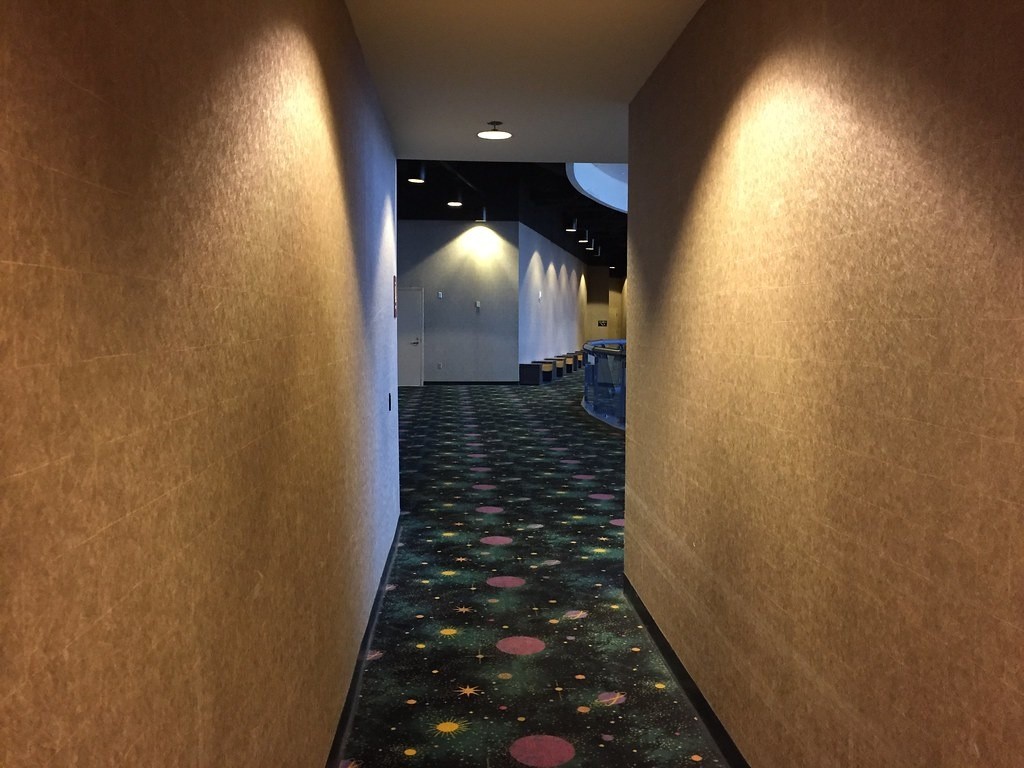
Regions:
[
  {"x1": 438, "y1": 362, "x2": 442, "y2": 369},
  {"x1": 475, "y1": 301, "x2": 481, "y2": 307},
  {"x1": 438, "y1": 291, "x2": 442, "y2": 298}
]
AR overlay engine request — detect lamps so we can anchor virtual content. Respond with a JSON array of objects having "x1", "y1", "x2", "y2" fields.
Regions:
[
  {"x1": 608, "y1": 262, "x2": 616, "y2": 269},
  {"x1": 565, "y1": 217, "x2": 578, "y2": 232},
  {"x1": 478, "y1": 120, "x2": 512, "y2": 140},
  {"x1": 447, "y1": 201, "x2": 463, "y2": 207},
  {"x1": 577, "y1": 229, "x2": 589, "y2": 244},
  {"x1": 592, "y1": 245, "x2": 600, "y2": 257},
  {"x1": 585, "y1": 238, "x2": 595, "y2": 251},
  {"x1": 408, "y1": 178, "x2": 425, "y2": 184}
]
